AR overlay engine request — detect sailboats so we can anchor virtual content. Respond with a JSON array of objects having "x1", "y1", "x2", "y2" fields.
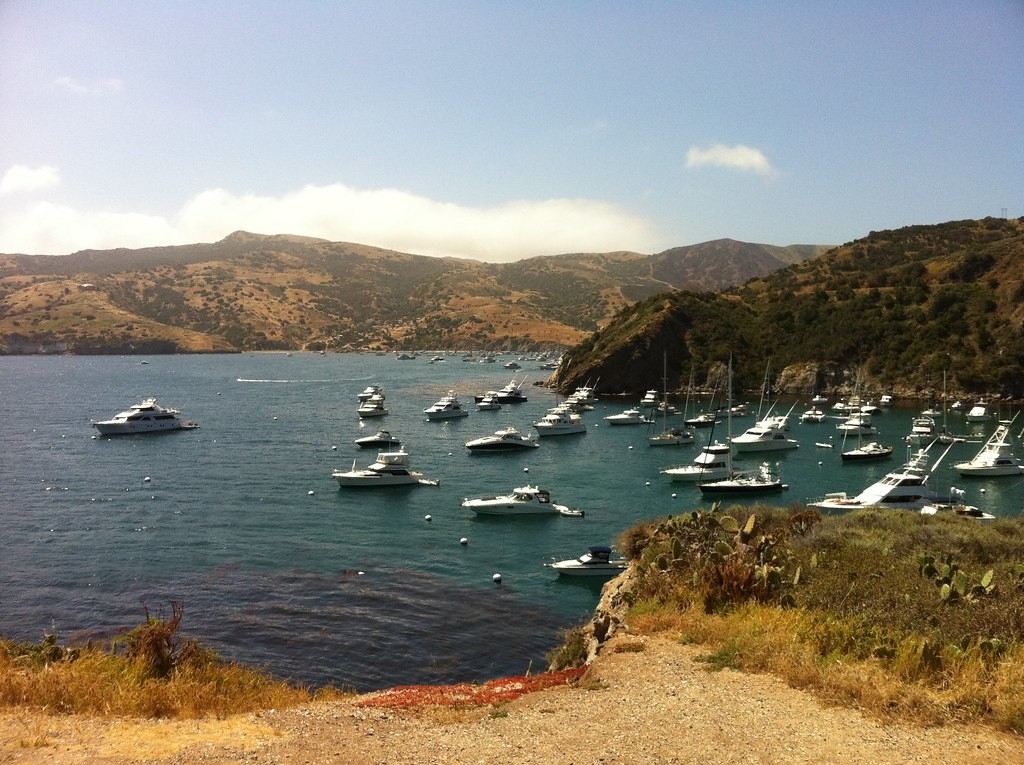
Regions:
[
  {"x1": 839, "y1": 355, "x2": 895, "y2": 461},
  {"x1": 697, "y1": 349, "x2": 783, "y2": 495}
]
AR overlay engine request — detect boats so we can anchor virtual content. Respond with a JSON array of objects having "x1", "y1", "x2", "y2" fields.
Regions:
[
  {"x1": 952, "y1": 410, "x2": 1024, "y2": 477},
  {"x1": 396, "y1": 349, "x2": 992, "y2": 453},
  {"x1": 417, "y1": 477, "x2": 440, "y2": 486},
  {"x1": 462, "y1": 484, "x2": 561, "y2": 515},
  {"x1": 918, "y1": 501, "x2": 997, "y2": 524},
  {"x1": 354, "y1": 429, "x2": 401, "y2": 449},
  {"x1": 560, "y1": 508, "x2": 585, "y2": 517},
  {"x1": 464, "y1": 426, "x2": 540, "y2": 452},
  {"x1": 356, "y1": 383, "x2": 386, "y2": 401},
  {"x1": 422, "y1": 387, "x2": 470, "y2": 420},
  {"x1": 805, "y1": 433, "x2": 959, "y2": 515},
  {"x1": 89, "y1": 397, "x2": 202, "y2": 436},
  {"x1": 356, "y1": 393, "x2": 389, "y2": 417},
  {"x1": 541, "y1": 545, "x2": 629, "y2": 576},
  {"x1": 330, "y1": 437, "x2": 425, "y2": 486}
]
